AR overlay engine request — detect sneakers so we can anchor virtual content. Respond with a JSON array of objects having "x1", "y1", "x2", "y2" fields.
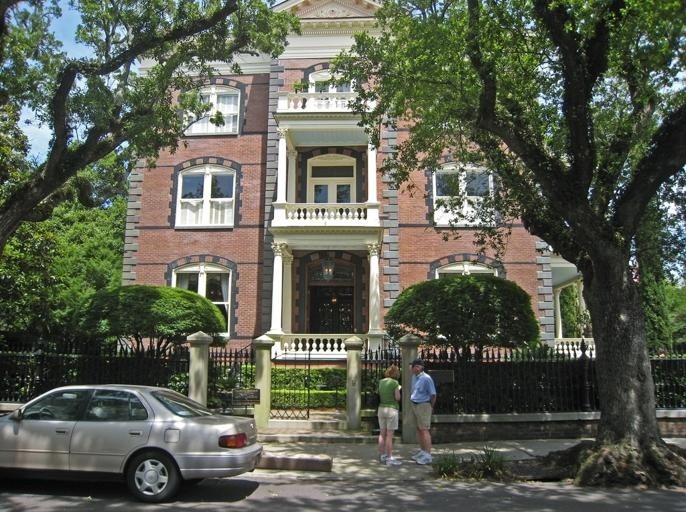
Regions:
[
  {"x1": 378, "y1": 454, "x2": 402, "y2": 466},
  {"x1": 410, "y1": 450, "x2": 432, "y2": 464}
]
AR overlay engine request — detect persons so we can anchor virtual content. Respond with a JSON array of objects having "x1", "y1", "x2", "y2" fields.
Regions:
[
  {"x1": 376, "y1": 363, "x2": 402, "y2": 466},
  {"x1": 407, "y1": 359, "x2": 438, "y2": 465}
]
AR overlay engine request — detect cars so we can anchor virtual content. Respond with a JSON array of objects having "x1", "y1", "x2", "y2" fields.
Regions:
[{"x1": 0, "y1": 382, "x2": 265, "y2": 504}]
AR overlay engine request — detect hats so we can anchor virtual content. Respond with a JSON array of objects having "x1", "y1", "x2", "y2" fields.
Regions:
[{"x1": 409, "y1": 359, "x2": 425, "y2": 366}]
